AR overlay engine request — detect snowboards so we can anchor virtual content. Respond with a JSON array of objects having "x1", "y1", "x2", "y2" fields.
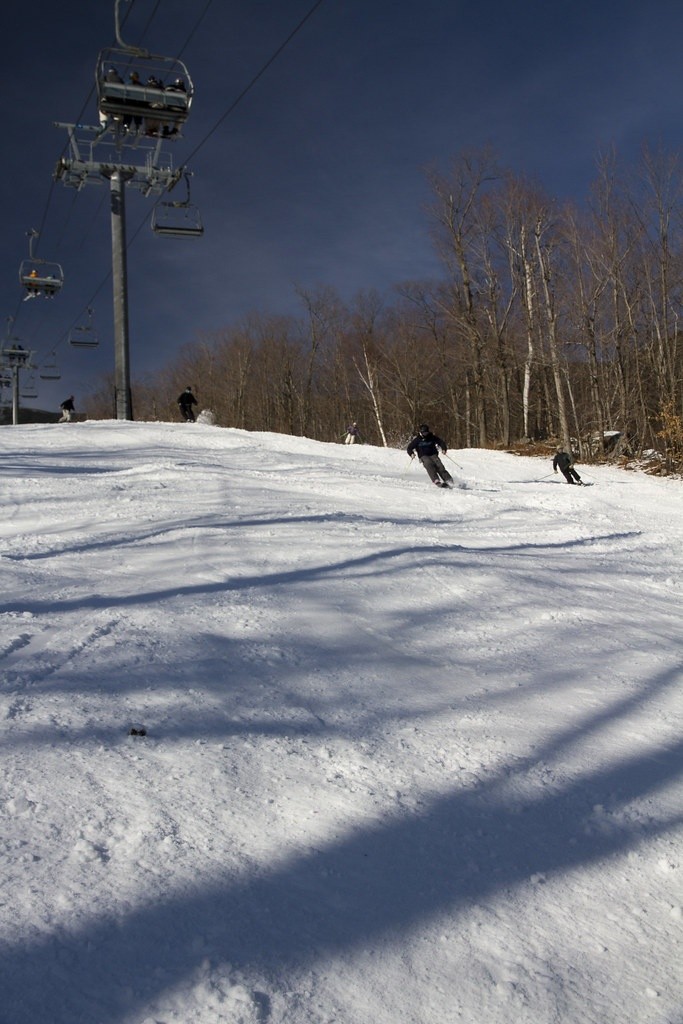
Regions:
[{"x1": 441, "y1": 482, "x2": 467, "y2": 489}]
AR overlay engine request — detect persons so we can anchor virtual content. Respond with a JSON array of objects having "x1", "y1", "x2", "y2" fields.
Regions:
[
  {"x1": 9, "y1": 344, "x2": 26, "y2": 364},
  {"x1": 44, "y1": 276, "x2": 55, "y2": 299},
  {"x1": 553, "y1": 445, "x2": 583, "y2": 484},
  {"x1": 59, "y1": 396, "x2": 75, "y2": 422},
  {"x1": 407, "y1": 424, "x2": 453, "y2": 487},
  {"x1": 0, "y1": 374, "x2": 11, "y2": 387},
  {"x1": 178, "y1": 387, "x2": 198, "y2": 423},
  {"x1": 345, "y1": 423, "x2": 360, "y2": 444},
  {"x1": 102, "y1": 67, "x2": 187, "y2": 141},
  {"x1": 26, "y1": 270, "x2": 38, "y2": 296}
]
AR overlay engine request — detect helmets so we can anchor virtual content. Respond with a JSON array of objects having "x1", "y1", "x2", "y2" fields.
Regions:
[{"x1": 420, "y1": 425, "x2": 428, "y2": 432}]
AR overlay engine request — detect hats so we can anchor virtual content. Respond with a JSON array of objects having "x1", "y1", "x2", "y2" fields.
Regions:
[{"x1": 556, "y1": 445, "x2": 564, "y2": 450}]
